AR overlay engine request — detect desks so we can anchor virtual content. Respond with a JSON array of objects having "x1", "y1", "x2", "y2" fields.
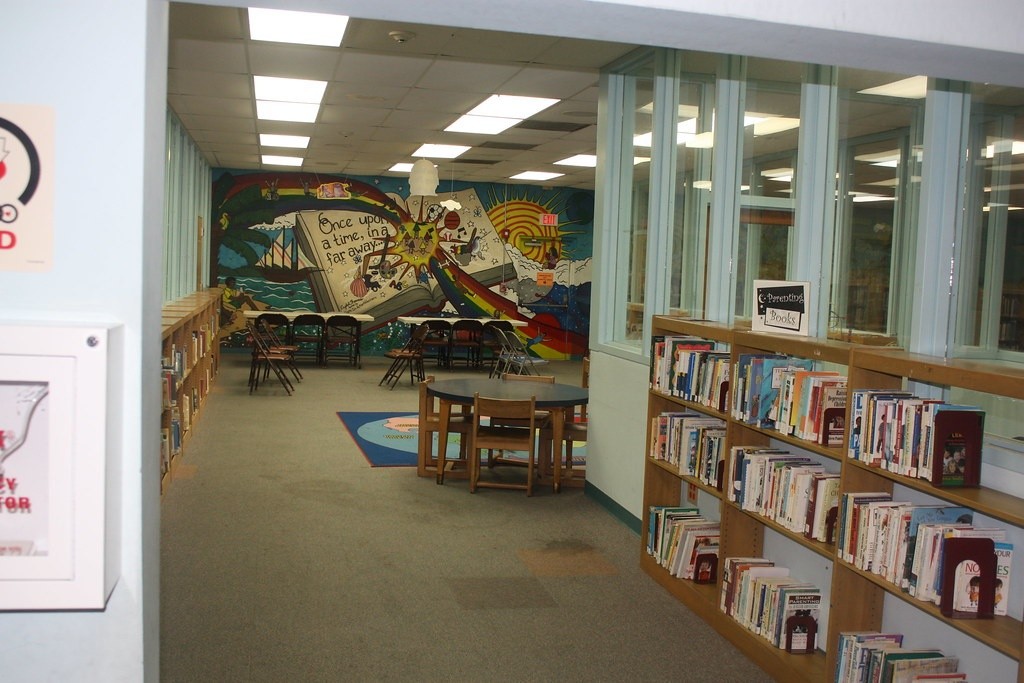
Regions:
[
  {"x1": 243, "y1": 310, "x2": 374, "y2": 355},
  {"x1": 428, "y1": 379, "x2": 589, "y2": 485},
  {"x1": 397, "y1": 316, "x2": 528, "y2": 339}
]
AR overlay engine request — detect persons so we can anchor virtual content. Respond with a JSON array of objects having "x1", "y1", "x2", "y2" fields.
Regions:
[
  {"x1": 953, "y1": 450, "x2": 961, "y2": 462},
  {"x1": 957, "y1": 458, "x2": 965, "y2": 473},
  {"x1": 876, "y1": 414, "x2": 885, "y2": 454},
  {"x1": 946, "y1": 460, "x2": 958, "y2": 474},
  {"x1": 854, "y1": 416, "x2": 861, "y2": 434},
  {"x1": 994, "y1": 578, "x2": 1002, "y2": 609},
  {"x1": 968, "y1": 576, "x2": 980, "y2": 606},
  {"x1": 943, "y1": 447, "x2": 953, "y2": 465},
  {"x1": 700, "y1": 562, "x2": 710, "y2": 579}
]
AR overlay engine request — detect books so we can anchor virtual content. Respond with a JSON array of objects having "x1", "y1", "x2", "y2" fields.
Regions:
[
  {"x1": 650, "y1": 412, "x2": 841, "y2": 542},
  {"x1": 848, "y1": 388, "x2": 986, "y2": 489},
  {"x1": 853, "y1": 288, "x2": 889, "y2": 332},
  {"x1": 1000, "y1": 296, "x2": 1022, "y2": 352},
  {"x1": 834, "y1": 631, "x2": 968, "y2": 683},
  {"x1": 837, "y1": 492, "x2": 1014, "y2": 616},
  {"x1": 648, "y1": 335, "x2": 847, "y2": 445},
  {"x1": 646, "y1": 506, "x2": 721, "y2": 581},
  {"x1": 161, "y1": 315, "x2": 214, "y2": 473},
  {"x1": 718, "y1": 557, "x2": 821, "y2": 650}
]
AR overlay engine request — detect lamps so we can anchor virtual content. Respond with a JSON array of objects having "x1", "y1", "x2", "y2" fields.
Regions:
[{"x1": 409, "y1": 157, "x2": 439, "y2": 197}]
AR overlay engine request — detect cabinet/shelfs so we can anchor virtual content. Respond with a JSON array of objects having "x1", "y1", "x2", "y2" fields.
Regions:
[
  {"x1": 161, "y1": 287, "x2": 223, "y2": 507},
  {"x1": 639, "y1": 315, "x2": 1023, "y2": 683}
]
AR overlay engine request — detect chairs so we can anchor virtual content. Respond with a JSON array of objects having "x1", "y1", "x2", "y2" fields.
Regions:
[
  {"x1": 418, "y1": 357, "x2": 590, "y2": 497},
  {"x1": 246, "y1": 313, "x2": 550, "y2": 397}
]
